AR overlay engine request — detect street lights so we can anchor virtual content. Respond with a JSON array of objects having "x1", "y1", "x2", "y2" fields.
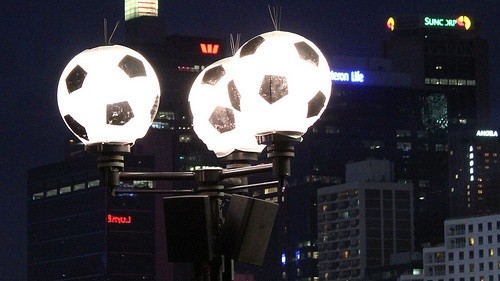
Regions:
[{"x1": 57, "y1": 30, "x2": 333, "y2": 281}]
